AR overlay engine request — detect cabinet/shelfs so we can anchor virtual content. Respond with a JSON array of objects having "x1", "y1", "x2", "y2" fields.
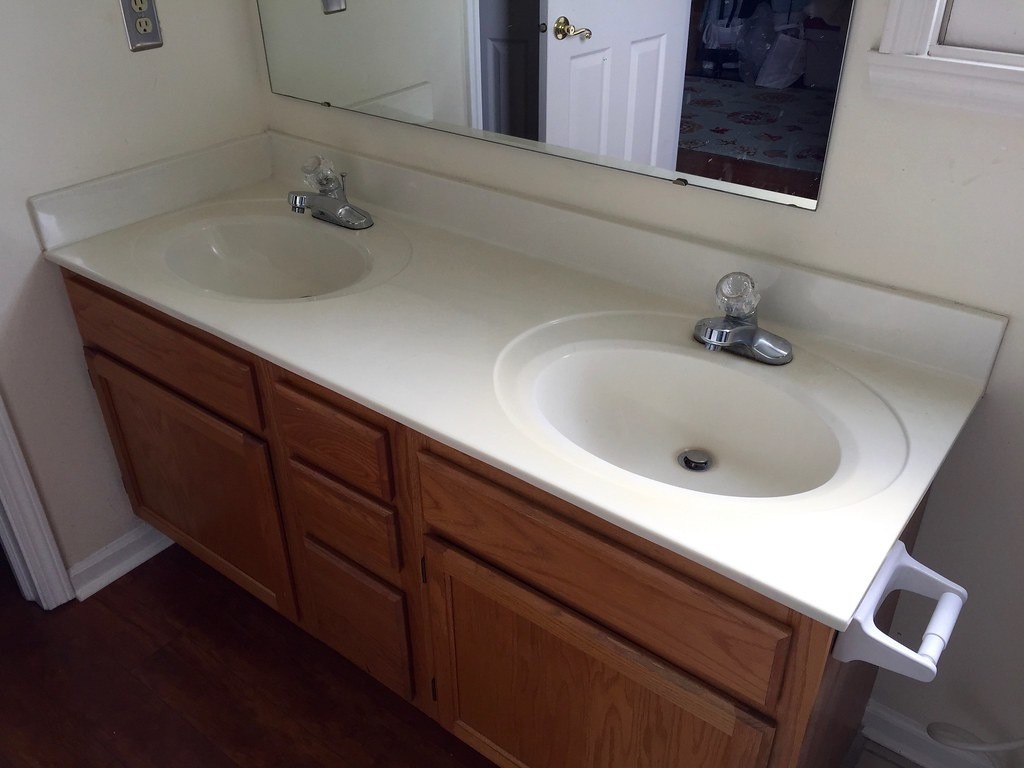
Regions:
[{"x1": 61, "y1": 267, "x2": 930, "y2": 768}]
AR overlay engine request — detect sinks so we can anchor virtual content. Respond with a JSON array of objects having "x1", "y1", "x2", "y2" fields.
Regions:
[
  {"x1": 161, "y1": 217, "x2": 378, "y2": 302},
  {"x1": 523, "y1": 339, "x2": 862, "y2": 497}
]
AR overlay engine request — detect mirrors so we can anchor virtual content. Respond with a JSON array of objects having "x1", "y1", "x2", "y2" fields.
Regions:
[{"x1": 256, "y1": 0, "x2": 856, "y2": 211}]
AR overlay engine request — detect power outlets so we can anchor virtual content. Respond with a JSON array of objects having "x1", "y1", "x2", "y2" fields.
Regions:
[{"x1": 119, "y1": 0, "x2": 163, "y2": 52}]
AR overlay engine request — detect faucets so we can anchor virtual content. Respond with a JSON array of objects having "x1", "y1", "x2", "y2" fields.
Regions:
[
  {"x1": 692, "y1": 272, "x2": 795, "y2": 366},
  {"x1": 288, "y1": 155, "x2": 372, "y2": 228}
]
